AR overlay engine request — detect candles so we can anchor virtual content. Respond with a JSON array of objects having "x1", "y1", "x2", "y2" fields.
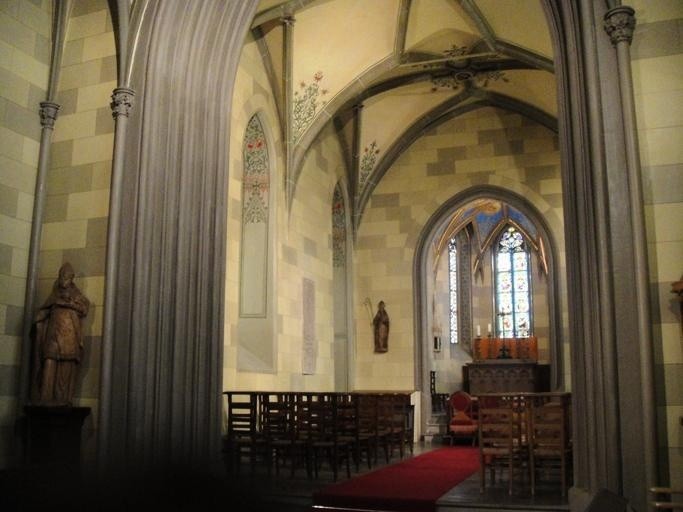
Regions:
[
  {"x1": 488, "y1": 323, "x2": 491, "y2": 332},
  {"x1": 477, "y1": 325, "x2": 480, "y2": 336}
]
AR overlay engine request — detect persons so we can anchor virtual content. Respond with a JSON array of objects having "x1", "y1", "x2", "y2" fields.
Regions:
[
  {"x1": 369, "y1": 299, "x2": 389, "y2": 352},
  {"x1": 26, "y1": 258, "x2": 92, "y2": 404}
]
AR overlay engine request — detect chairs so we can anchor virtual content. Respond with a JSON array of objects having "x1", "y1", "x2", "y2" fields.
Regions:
[
  {"x1": 443, "y1": 391, "x2": 480, "y2": 447},
  {"x1": 223, "y1": 392, "x2": 414, "y2": 482},
  {"x1": 430, "y1": 371, "x2": 449, "y2": 411},
  {"x1": 471, "y1": 392, "x2": 571, "y2": 497}
]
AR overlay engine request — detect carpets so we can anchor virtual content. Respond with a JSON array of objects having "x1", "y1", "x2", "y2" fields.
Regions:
[{"x1": 305, "y1": 446, "x2": 503, "y2": 512}]
